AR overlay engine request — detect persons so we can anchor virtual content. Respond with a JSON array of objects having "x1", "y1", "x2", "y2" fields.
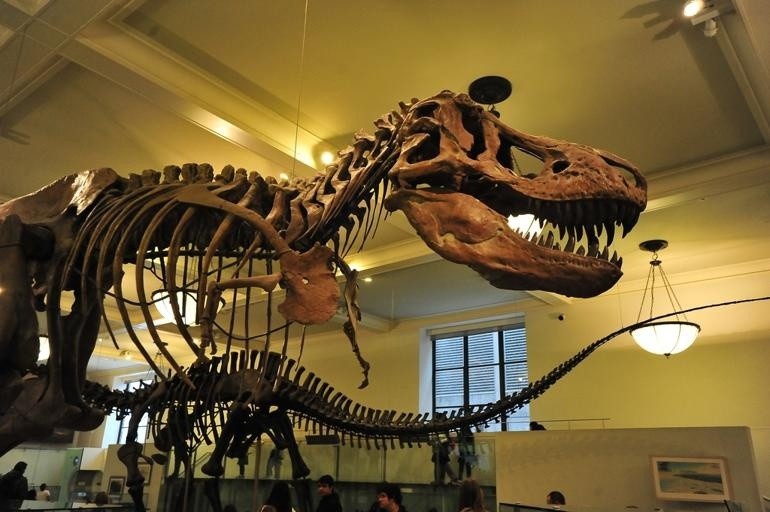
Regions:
[
  {"x1": 377, "y1": 483, "x2": 407, "y2": 512},
  {"x1": 36, "y1": 484, "x2": 50, "y2": 501},
  {"x1": 530, "y1": 422, "x2": 537, "y2": 430},
  {"x1": 547, "y1": 491, "x2": 565, "y2": 504},
  {"x1": 428, "y1": 412, "x2": 457, "y2": 485},
  {"x1": 169, "y1": 441, "x2": 188, "y2": 477},
  {"x1": 0, "y1": 462, "x2": 36, "y2": 512},
  {"x1": 266, "y1": 445, "x2": 283, "y2": 480},
  {"x1": 459, "y1": 480, "x2": 481, "y2": 512},
  {"x1": 316, "y1": 475, "x2": 342, "y2": 512},
  {"x1": 458, "y1": 424, "x2": 474, "y2": 483},
  {"x1": 534, "y1": 425, "x2": 546, "y2": 430},
  {"x1": 264, "y1": 480, "x2": 296, "y2": 512}
]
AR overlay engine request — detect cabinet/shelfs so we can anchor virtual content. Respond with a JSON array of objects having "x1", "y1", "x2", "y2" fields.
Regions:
[{"x1": 161, "y1": 438, "x2": 496, "y2": 512}]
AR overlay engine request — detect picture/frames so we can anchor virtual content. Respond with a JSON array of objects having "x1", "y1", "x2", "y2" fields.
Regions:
[
  {"x1": 108, "y1": 476, "x2": 125, "y2": 495},
  {"x1": 137, "y1": 462, "x2": 152, "y2": 484},
  {"x1": 651, "y1": 457, "x2": 732, "y2": 503}
]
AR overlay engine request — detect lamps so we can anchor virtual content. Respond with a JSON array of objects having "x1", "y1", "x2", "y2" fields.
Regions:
[
  {"x1": 630, "y1": 241, "x2": 700, "y2": 356},
  {"x1": 151, "y1": 289, "x2": 224, "y2": 328}
]
